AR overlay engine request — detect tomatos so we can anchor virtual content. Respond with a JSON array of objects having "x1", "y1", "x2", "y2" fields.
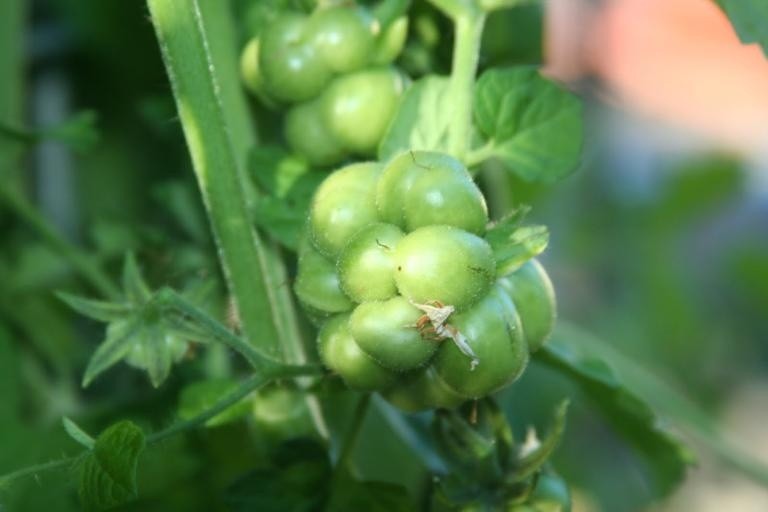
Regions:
[{"x1": 239, "y1": 8, "x2": 555, "y2": 413}]
